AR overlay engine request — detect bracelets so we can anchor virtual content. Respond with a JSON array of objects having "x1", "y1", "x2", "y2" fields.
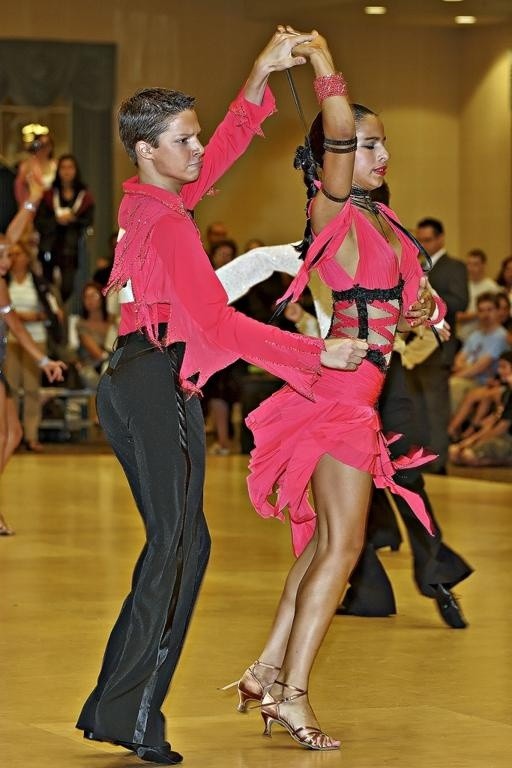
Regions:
[{"x1": 312, "y1": 72, "x2": 348, "y2": 106}]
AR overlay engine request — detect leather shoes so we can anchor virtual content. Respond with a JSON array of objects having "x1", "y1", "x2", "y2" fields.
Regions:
[
  {"x1": 85, "y1": 730, "x2": 183, "y2": 764},
  {"x1": 435, "y1": 583, "x2": 466, "y2": 627}
]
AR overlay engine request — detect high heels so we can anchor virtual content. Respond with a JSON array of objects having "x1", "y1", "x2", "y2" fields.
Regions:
[
  {"x1": 219, "y1": 661, "x2": 280, "y2": 712},
  {"x1": 249, "y1": 680, "x2": 339, "y2": 750}
]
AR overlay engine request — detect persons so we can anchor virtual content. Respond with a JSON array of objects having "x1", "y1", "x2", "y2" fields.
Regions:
[
  {"x1": 74, "y1": 26, "x2": 369, "y2": 766},
  {"x1": 215, "y1": 23, "x2": 452, "y2": 753}
]
